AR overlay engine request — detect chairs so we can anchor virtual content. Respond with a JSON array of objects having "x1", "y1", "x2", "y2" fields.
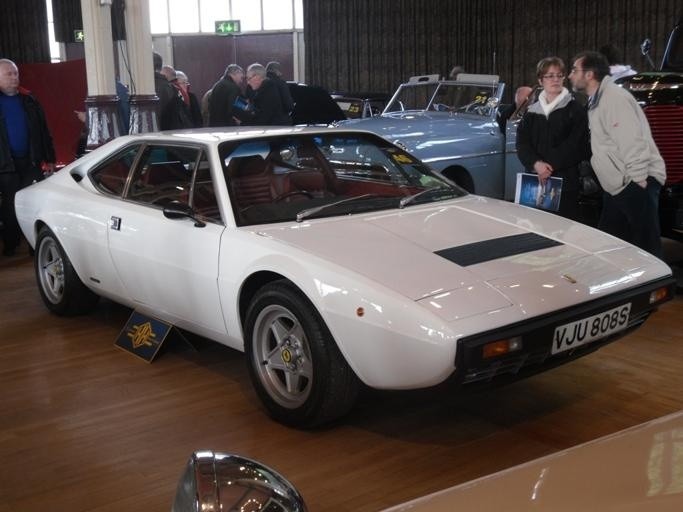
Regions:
[{"x1": 225, "y1": 156, "x2": 283, "y2": 212}]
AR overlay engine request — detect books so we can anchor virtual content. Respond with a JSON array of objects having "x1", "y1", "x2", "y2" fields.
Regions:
[{"x1": 515, "y1": 171, "x2": 563, "y2": 213}]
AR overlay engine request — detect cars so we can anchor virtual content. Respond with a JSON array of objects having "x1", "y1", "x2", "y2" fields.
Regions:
[
  {"x1": 14, "y1": 124, "x2": 674, "y2": 428},
  {"x1": 286, "y1": 78, "x2": 392, "y2": 119},
  {"x1": 172, "y1": 409, "x2": 683, "y2": 512},
  {"x1": 280, "y1": 66, "x2": 542, "y2": 203}
]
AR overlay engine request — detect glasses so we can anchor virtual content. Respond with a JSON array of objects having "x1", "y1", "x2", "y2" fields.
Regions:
[{"x1": 544, "y1": 73, "x2": 566, "y2": 80}]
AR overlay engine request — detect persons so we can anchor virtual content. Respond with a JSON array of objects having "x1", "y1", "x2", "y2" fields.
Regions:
[
  {"x1": 499, "y1": 86, "x2": 535, "y2": 138},
  {"x1": 0, "y1": 60, "x2": 54, "y2": 257},
  {"x1": 567, "y1": 50, "x2": 666, "y2": 260},
  {"x1": 514, "y1": 57, "x2": 589, "y2": 220},
  {"x1": 150, "y1": 53, "x2": 297, "y2": 131}
]
